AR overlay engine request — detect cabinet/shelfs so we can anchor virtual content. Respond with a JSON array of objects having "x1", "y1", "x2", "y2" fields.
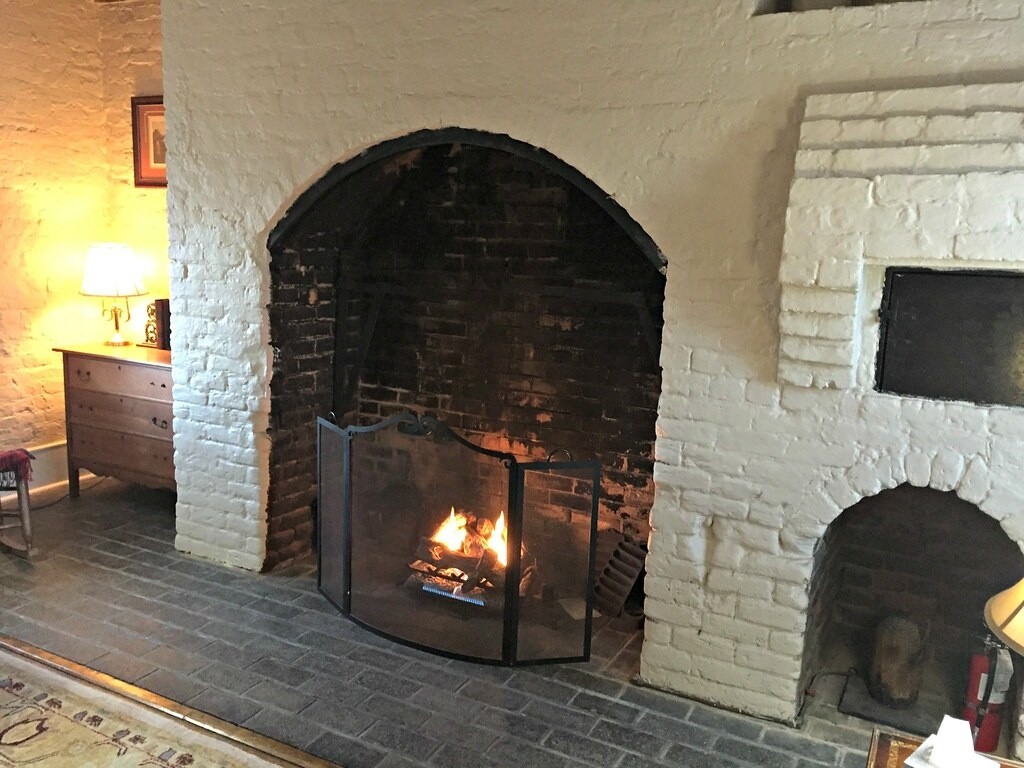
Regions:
[{"x1": 51, "y1": 336, "x2": 178, "y2": 500}]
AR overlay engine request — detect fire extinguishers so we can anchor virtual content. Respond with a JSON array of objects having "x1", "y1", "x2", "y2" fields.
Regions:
[{"x1": 965, "y1": 633, "x2": 1008, "y2": 753}]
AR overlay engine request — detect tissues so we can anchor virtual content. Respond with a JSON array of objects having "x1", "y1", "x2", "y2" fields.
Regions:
[{"x1": 904, "y1": 714, "x2": 1001, "y2": 768}]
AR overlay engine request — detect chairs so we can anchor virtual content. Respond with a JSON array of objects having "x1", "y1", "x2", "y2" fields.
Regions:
[{"x1": 0, "y1": 445, "x2": 41, "y2": 561}]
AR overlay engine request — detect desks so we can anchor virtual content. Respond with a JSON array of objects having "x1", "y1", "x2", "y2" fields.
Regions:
[{"x1": 867, "y1": 724, "x2": 1024, "y2": 768}]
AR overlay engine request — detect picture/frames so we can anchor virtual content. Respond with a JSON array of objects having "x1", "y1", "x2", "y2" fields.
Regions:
[
  {"x1": 131, "y1": 93, "x2": 169, "y2": 189},
  {"x1": 79, "y1": 243, "x2": 151, "y2": 347}
]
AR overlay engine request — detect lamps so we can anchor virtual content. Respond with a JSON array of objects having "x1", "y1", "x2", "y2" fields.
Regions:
[{"x1": 983, "y1": 576, "x2": 1024, "y2": 658}]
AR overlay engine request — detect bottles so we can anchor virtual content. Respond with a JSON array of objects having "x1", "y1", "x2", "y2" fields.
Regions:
[{"x1": 155, "y1": 298, "x2": 170, "y2": 350}]
